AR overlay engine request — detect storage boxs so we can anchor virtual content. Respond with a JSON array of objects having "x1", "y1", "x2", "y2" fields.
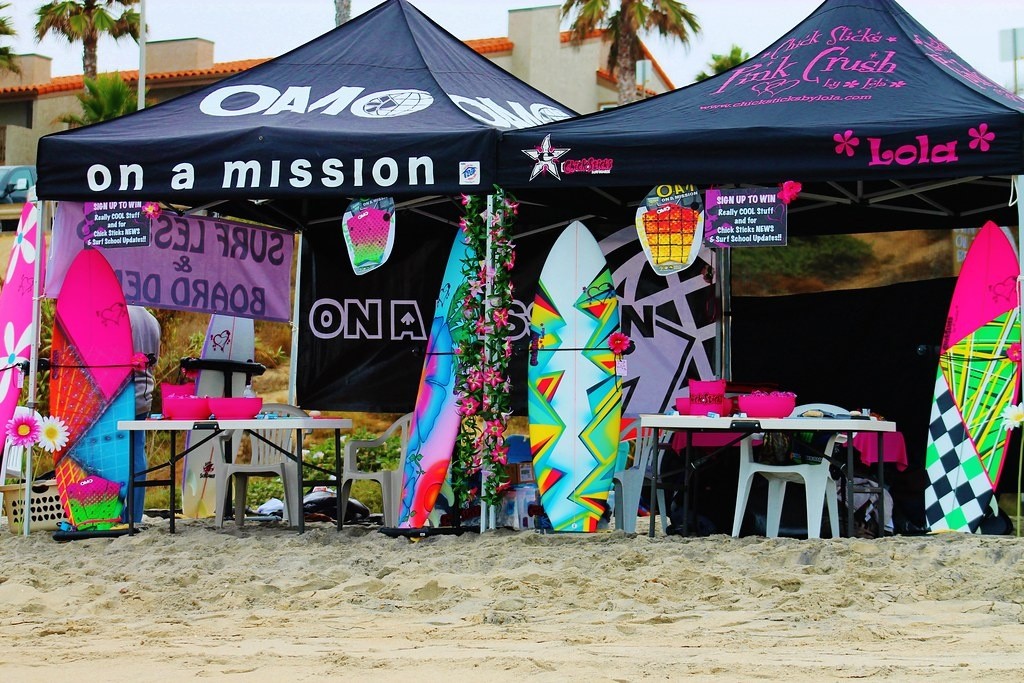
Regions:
[
  {"x1": 0, "y1": 478, "x2": 69, "y2": 534},
  {"x1": 502, "y1": 461, "x2": 545, "y2": 531}
]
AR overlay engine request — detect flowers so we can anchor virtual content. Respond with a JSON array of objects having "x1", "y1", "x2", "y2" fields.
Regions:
[
  {"x1": 608, "y1": 332, "x2": 630, "y2": 355},
  {"x1": 4, "y1": 412, "x2": 71, "y2": 454},
  {"x1": 1006, "y1": 343, "x2": 1022, "y2": 361},
  {"x1": 450, "y1": 192, "x2": 521, "y2": 504},
  {"x1": 1000, "y1": 402, "x2": 1024, "y2": 536},
  {"x1": 777, "y1": 180, "x2": 803, "y2": 204}
]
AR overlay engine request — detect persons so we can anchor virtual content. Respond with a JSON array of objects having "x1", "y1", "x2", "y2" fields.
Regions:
[{"x1": 126, "y1": 306, "x2": 161, "y2": 523}]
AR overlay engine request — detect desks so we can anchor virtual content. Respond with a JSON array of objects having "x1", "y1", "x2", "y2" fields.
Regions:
[
  {"x1": 117, "y1": 418, "x2": 352, "y2": 539},
  {"x1": 638, "y1": 413, "x2": 896, "y2": 543}
]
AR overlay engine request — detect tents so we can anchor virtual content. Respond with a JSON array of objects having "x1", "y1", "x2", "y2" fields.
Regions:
[
  {"x1": 24, "y1": 0, "x2": 722, "y2": 537},
  {"x1": 490, "y1": 0, "x2": 1023, "y2": 536}
]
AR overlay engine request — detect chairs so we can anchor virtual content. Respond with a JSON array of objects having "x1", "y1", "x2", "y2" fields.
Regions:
[
  {"x1": 212, "y1": 403, "x2": 314, "y2": 527},
  {"x1": 730, "y1": 403, "x2": 857, "y2": 544},
  {"x1": 0, "y1": 404, "x2": 41, "y2": 517},
  {"x1": 340, "y1": 412, "x2": 415, "y2": 529},
  {"x1": 607, "y1": 417, "x2": 676, "y2": 536},
  {"x1": 499, "y1": 433, "x2": 540, "y2": 531}
]
